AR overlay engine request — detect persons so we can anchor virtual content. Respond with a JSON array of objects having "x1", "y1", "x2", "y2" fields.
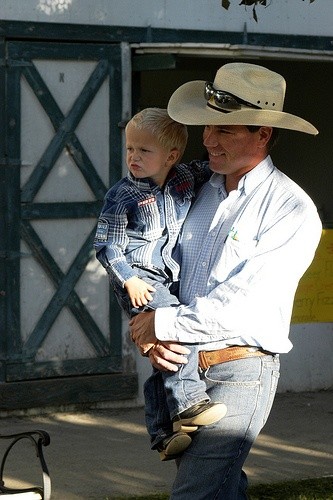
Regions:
[
  {"x1": 124, "y1": 62, "x2": 323, "y2": 500},
  {"x1": 90, "y1": 106, "x2": 226, "y2": 460}
]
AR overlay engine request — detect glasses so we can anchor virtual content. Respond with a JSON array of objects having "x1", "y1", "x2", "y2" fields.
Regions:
[{"x1": 204, "y1": 82, "x2": 262, "y2": 110}]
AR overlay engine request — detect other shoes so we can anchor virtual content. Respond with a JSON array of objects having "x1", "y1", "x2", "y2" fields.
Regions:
[
  {"x1": 171, "y1": 401, "x2": 227, "y2": 433},
  {"x1": 157, "y1": 431, "x2": 192, "y2": 461}
]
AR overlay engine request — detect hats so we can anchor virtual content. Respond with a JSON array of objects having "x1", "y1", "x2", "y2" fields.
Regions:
[{"x1": 167, "y1": 62, "x2": 319, "y2": 135}]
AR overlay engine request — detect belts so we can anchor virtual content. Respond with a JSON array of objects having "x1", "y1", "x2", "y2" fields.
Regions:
[{"x1": 198, "y1": 345, "x2": 268, "y2": 372}]
